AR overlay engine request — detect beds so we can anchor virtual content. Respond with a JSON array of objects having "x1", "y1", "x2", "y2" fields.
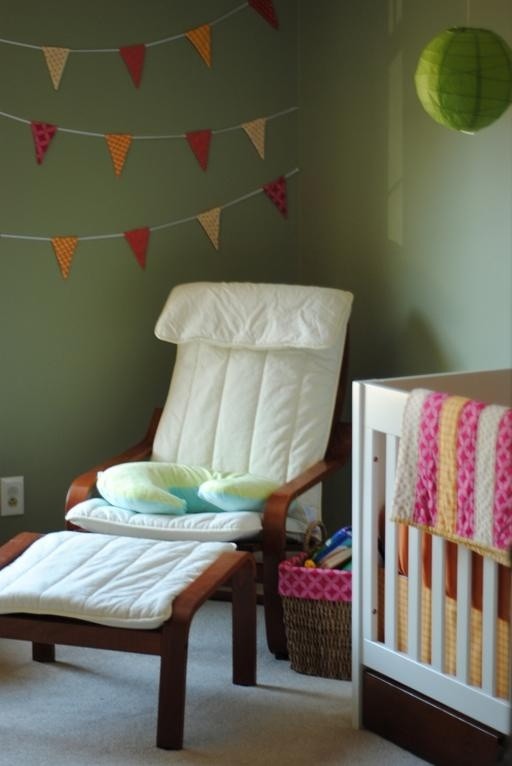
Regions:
[{"x1": 350, "y1": 365, "x2": 512, "y2": 765}]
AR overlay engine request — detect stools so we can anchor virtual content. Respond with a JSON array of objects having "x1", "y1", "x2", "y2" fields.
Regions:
[{"x1": 1, "y1": 530, "x2": 258, "y2": 750}]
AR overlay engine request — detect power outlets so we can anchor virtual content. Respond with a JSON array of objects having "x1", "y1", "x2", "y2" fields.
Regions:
[{"x1": 0, "y1": 475, "x2": 24, "y2": 516}]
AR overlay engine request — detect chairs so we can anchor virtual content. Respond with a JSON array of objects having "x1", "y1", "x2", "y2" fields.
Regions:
[{"x1": 64, "y1": 281, "x2": 354, "y2": 658}]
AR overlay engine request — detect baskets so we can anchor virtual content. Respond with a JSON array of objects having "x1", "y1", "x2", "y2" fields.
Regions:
[{"x1": 278, "y1": 521, "x2": 351, "y2": 680}]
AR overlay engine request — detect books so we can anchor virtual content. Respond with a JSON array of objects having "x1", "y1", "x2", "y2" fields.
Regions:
[{"x1": 312, "y1": 525, "x2": 352, "y2": 572}]
